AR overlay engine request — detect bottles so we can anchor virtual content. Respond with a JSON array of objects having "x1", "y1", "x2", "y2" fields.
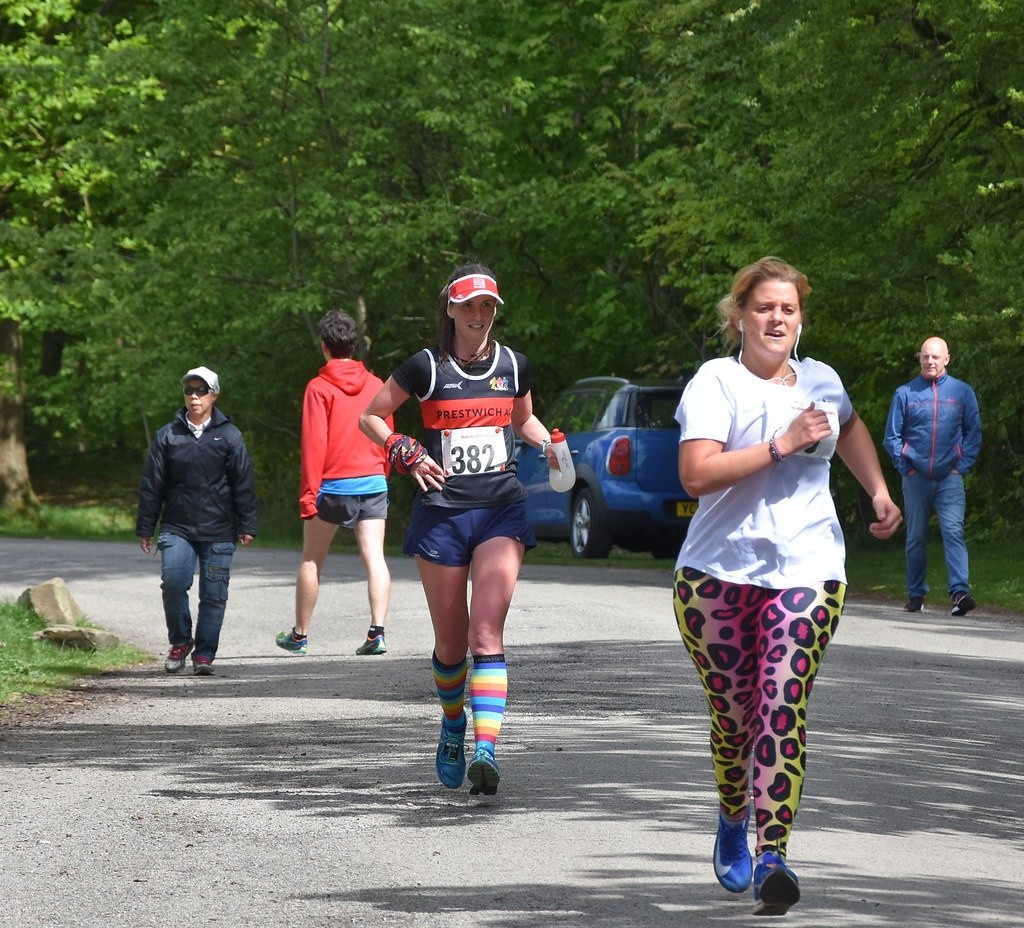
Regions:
[{"x1": 549, "y1": 428, "x2": 576, "y2": 492}]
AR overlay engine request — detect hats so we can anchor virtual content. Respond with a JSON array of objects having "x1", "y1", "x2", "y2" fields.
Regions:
[
  {"x1": 445, "y1": 274, "x2": 505, "y2": 319},
  {"x1": 180, "y1": 365, "x2": 219, "y2": 394}
]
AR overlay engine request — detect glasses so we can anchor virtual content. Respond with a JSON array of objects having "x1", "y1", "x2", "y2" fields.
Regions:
[{"x1": 182, "y1": 384, "x2": 215, "y2": 396}]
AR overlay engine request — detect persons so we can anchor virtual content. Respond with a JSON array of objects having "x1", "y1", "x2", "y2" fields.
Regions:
[
  {"x1": 672, "y1": 255, "x2": 904, "y2": 915},
  {"x1": 357, "y1": 264, "x2": 560, "y2": 796},
  {"x1": 136, "y1": 366, "x2": 258, "y2": 675},
  {"x1": 276, "y1": 307, "x2": 395, "y2": 656},
  {"x1": 883, "y1": 336, "x2": 982, "y2": 617}
]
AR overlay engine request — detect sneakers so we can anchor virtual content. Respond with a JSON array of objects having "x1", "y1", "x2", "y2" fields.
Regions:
[
  {"x1": 713, "y1": 808, "x2": 754, "y2": 893},
  {"x1": 906, "y1": 596, "x2": 925, "y2": 612},
  {"x1": 467, "y1": 743, "x2": 500, "y2": 797},
  {"x1": 356, "y1": 635, "x2": 387, "y2": 655},
  {"x1": 192, "y1": 655, "x2": 215, "y2": 675},
  {"x1": 750, "y1": 852, "x2": 801, "y2": 917},
  {"x1": 276, "y1": 631, "x2": 308, "y2": 655},
  {"x1": 165, "y1": 638, "x2": 194, "y2": 672},
  {"x1": 951, "y1": 596, "x2": 975, "y2": 615},
  {"x1": 435, "y1": 712, "x2": 468, "y2": 789}
]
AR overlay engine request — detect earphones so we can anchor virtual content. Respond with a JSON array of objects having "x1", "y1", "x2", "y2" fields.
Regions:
[
  {"x1": 739, "y1": 319, "x2": 743, "y2": 331},
  {"x1": 797, "y1": 324, "x2": 802, "y2": 335}
]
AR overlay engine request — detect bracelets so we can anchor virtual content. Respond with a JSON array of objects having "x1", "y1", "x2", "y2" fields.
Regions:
[
  {"x1": 770, "y1": 439, "x2": 784, "y2": 461},
  {"x1": 384, "y1": 432, "x2": 427, "y2": 474},
  {"x1": 539, "y1": 439, "x2": 551, "y2": 456}
]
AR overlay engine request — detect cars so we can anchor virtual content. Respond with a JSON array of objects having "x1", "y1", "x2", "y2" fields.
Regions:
[{"x1": 515, "y1": 374, "x2": 700, "y2": 561}]
